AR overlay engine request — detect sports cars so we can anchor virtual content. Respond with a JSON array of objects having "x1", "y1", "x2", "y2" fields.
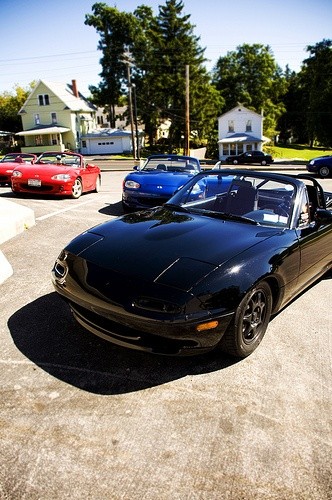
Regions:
[
  {"x1": 11, "y1": 151, "x2": 102, "y2": 199},
  {"x1": 121, "y1": 155, "x2": 238, "y2": 213},
  {"x1": 50, "y1": 169, "x2": 332, "y2": 359},
  {"x1": 0, "y1": 153, "x2": 38, "y2": 188}
]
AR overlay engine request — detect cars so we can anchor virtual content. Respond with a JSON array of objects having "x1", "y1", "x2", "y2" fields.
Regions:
[
  {"x1": 224, "y1": 150, "x2": 274, "y2": 165},
  {"x1": 306, "y1": 155, "x2": 332, "y2": 177}
]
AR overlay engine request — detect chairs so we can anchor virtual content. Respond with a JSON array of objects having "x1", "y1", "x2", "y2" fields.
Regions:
[
  {"x1": 185, "y1": 164, "x2": 195, "y2": 170},
  {"x1": 230, "y1": 185, "x2": 255, "y2": 215},
  {"x1": 305, "y1": 185, "x2": 318, "y2": 219},
  {"x1": 157, "y1": 164, "x2": 166, "y2": 171}
]
